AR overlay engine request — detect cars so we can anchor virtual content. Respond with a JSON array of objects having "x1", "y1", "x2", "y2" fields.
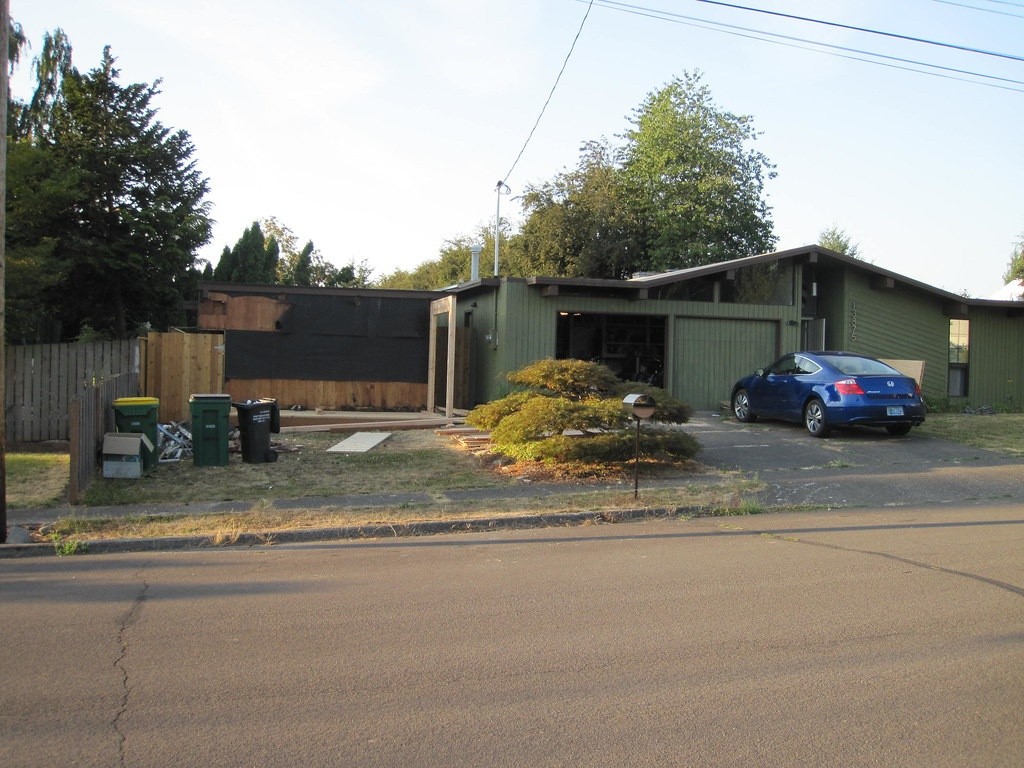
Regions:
[{"x1": 730, "y1": 350, "x2": 927, "y2": 439}]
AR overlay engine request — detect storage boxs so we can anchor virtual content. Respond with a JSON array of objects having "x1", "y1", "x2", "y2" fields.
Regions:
[{"x1": 102, "y1": 433, "x2": 155, "y2": 478}]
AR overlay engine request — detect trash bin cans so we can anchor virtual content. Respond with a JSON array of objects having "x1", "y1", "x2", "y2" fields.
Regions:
[
  {"x1": 111, "y1": 396, "x2": 161, "y2": 469},
  {"x1": 188, "y1": 394, "x2": 230, "y2": 467},
  {"x1": 229, "y1": 397, "x2": 281, "y2": 464}
]
